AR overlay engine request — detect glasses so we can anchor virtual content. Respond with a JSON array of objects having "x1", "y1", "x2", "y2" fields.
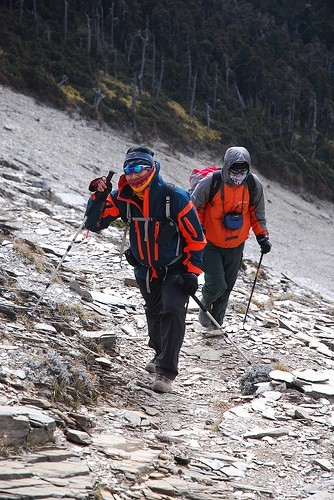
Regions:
[
  {"x1": 124, "y1": 165, "x2": 151, "y2": 175},
  {"x1": 229, "y1": 169, "x2": 248, "y2": 175}
]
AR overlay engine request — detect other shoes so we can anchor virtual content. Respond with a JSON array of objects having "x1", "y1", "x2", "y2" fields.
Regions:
[{"x1": 199, "y1": 298, "x2": 211, "y2": 326}]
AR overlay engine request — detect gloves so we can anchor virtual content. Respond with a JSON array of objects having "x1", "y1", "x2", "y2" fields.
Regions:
[
  {"x1": 179, "y1": 272, "x2": 199, "y2": 296},
  {"x1": 258, "y1": 236, "x2": 272, "y2": 254}
]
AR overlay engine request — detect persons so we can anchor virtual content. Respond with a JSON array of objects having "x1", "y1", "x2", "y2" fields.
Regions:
[
  {"x1": 84, "y1": 145, "x2": 207, "y2": 392},
  {"x1": 191, "y1": 147, "x2": 272, "y2": 330}
]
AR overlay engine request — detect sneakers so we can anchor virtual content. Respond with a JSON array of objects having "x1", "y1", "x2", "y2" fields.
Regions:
[
  {"x1": 153, "y1": 374, "x2": 171, "y2": 393},
  {"x1": 145, "y1": 358, "x2": 157, "y2": 373}
]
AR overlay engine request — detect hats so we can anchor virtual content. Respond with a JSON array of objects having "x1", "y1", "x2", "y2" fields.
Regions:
[
  {"x1": 230, "y1": 161, "x2": 249, "y2": 169},
  {"x1": 123, "y1": 146, "x2": 155, "y2": 169}
]
